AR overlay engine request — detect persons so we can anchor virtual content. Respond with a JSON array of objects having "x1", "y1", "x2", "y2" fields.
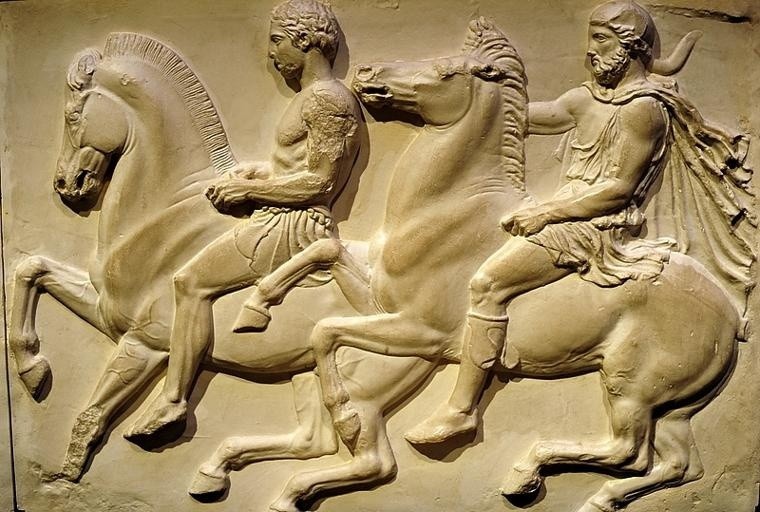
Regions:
[
  {"x1": 401, "y1": 0, "x2": 672, "y2": 445},
  {"x1": 122, "y1": 0, "x2": 365, "y2": 439}
]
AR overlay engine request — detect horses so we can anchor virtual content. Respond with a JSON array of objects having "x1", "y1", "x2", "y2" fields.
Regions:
[
  {"x1": 8, "y1": 31, "x2": 448, "y2": 511},
  {"x1": 232, "y1": 15, "x2": 751, "y2": 512}
]
